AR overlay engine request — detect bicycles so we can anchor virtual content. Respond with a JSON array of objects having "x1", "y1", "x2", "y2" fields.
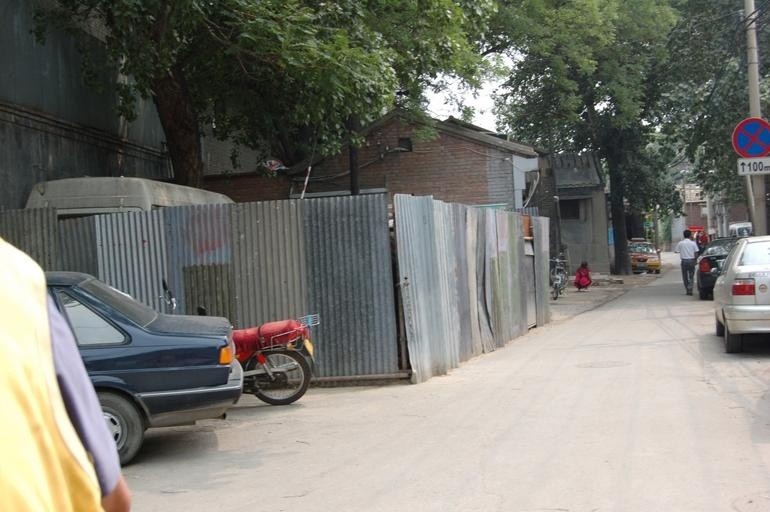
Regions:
[{"x1": 546, "y1": 253, "x2": 572, "y2": 301}]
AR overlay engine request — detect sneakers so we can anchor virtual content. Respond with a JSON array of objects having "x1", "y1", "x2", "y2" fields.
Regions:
[{"x1": 686, "y1": 288, "x2": 693, "y2": 295}]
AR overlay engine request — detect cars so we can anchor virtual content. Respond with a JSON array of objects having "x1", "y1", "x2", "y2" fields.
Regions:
[
  {"x1": 695, "y1": 221, "x2": 754, "y2": 301},
  {"x1": 712, "y1": 233, "x2": 770, "y2": 354},
  {"x1": 625, "y1": 237, "x2": 665, "y2": 277},
  {"x1": 38, "y1": 270, "x2": 249, "y2": 469}
]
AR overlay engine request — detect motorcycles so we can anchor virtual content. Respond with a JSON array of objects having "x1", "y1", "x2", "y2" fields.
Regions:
[{"x1": 154, "y1": 277, "x2": 325, "y2": 412}]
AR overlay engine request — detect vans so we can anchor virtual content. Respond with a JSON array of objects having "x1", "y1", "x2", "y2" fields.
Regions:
[{"x1": 20, "y1": 175, "x2": 241, "y2": 223}]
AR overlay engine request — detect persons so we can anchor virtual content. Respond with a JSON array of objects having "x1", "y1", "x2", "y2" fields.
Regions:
[
  {"x1": 675, "y1": 230, "x2": 701, "y2": 294},
  {"x1": 1, "y1": 239, "x2": 132, "y2": 511},
  {"x1": 574, "y1": 261, "x2": 591, "y2": 289}
]
